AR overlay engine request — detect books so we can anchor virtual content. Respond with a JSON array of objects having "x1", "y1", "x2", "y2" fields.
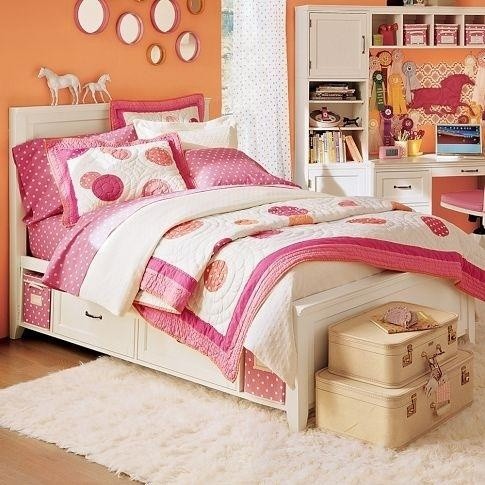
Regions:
[
  {"x1": 370, "y1": 312, "x2": 438, "y2": 333},
  {"x1": 310, "y1": 84, "x2": 357, "y2": 101},
  {"x1": 309, "y1": 131, "x2": 363, "y2": 164}
]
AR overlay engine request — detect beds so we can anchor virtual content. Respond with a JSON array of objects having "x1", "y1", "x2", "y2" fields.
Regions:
[{"x1": 4, "y1": 97, "x2": 478, "y2": 436}]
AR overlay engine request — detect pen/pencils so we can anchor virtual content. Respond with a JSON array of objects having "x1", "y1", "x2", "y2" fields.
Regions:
[{"x1": 390, "y1": 130, "x2": 408, "y2": 141}]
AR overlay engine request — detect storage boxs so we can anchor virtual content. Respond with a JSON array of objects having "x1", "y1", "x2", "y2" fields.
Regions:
[
  {"x1": 435, "y1": 22, "x2": 461, "y2": 47},
  {"x1": 403, "y1": 22, "x2": 430, "y2": 47},
  {"x1": 238, "y1": 344, "x2": 288, "y2": 408},
  {"x1": 465, "y1": 23, "x2": 485, "y2": 46},
  {"x1": 20, "y1": 269, "x2": 53, "y2": 331}
]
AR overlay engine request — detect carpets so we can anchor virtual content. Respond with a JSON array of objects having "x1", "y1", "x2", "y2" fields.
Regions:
[{"x1": 0, "y1": 296, "x2": 485, "y2": 485}]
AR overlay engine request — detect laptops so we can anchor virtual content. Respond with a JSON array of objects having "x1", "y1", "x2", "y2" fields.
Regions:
[{"x1": 435, "y1": 124, "x2": 485, "y2": 162}]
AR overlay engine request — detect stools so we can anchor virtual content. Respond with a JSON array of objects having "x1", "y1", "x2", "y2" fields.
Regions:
[{"x1": 438, "y1": 188, "x2": 484, "y2": 237}]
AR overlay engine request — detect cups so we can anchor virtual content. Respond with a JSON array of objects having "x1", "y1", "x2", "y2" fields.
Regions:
[
  {"x1": 379, "y1": 23, "x2": 396, "y2": 45},
  {"x1": 373, "y1": 34, "x2": 383, "y2": 44},
  {"x1": 394, "y1": 140, "x2": 408, "y2": 158},
  {"x1": 408, "y1": 138, "x2": 423, "y2": 153}
]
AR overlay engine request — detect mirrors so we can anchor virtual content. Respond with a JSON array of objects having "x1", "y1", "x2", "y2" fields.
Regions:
[
  {"x1": 186, "y1": 0, "x2": 205, "y2": 15},
  {"x1": 73, "y1": 0, "x2": 110, "y2": 35},
  {"x1": 175, "y1": 29, "x2": 202, "y2": 64},
  {"x1": 145, "y1": 42, "x2": 167, "y2": 67},
  {"x1": 115, "y1": 11, "x2": 145, "y2": 47},
  {"x1": 149, "y1": 0, "x2": 182, "y2": 36}
]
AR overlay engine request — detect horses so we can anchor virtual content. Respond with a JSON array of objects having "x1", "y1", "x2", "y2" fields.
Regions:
[
  {"x1": 37, "y1": 66, "x2": 81, "y2": 106},
  {"x1": 81, "y1": 74, "x2": 113, "y2": 103}
]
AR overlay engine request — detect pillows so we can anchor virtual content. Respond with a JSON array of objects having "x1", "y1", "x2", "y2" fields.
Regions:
[
  {"x1": 129, "y1": 111, "x2": 238, "y2": 152},
  {"x1": 183, "y1": 147, "x2": 304, "y2": 194},
  {"x1": 45, "y1": 131, "x2": 195, "y2": 229},
  {"x1": 107, "y1": 92, "x2": 206, "y2": 132},
  {"x1": 12, "y1": 123, "x2": 141, "y2": 227}
]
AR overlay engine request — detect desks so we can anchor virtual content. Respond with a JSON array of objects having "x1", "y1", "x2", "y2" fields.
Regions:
[{"x1": 368, "y1": 152, "x2": 485, "y2": 217}]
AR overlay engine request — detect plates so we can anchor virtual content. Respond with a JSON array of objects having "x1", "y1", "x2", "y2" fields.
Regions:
[{"x1": 408, "y1": 152, "x2": 424, "y2": 156}]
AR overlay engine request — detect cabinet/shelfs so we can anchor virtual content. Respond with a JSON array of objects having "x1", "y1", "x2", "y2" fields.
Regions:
[
  {"x1": 292, "y1": 4, "x2": 371, "y2": 198},
  {"x1": 367, "y1": 4, "x2": 485, "y2": 52}
]
